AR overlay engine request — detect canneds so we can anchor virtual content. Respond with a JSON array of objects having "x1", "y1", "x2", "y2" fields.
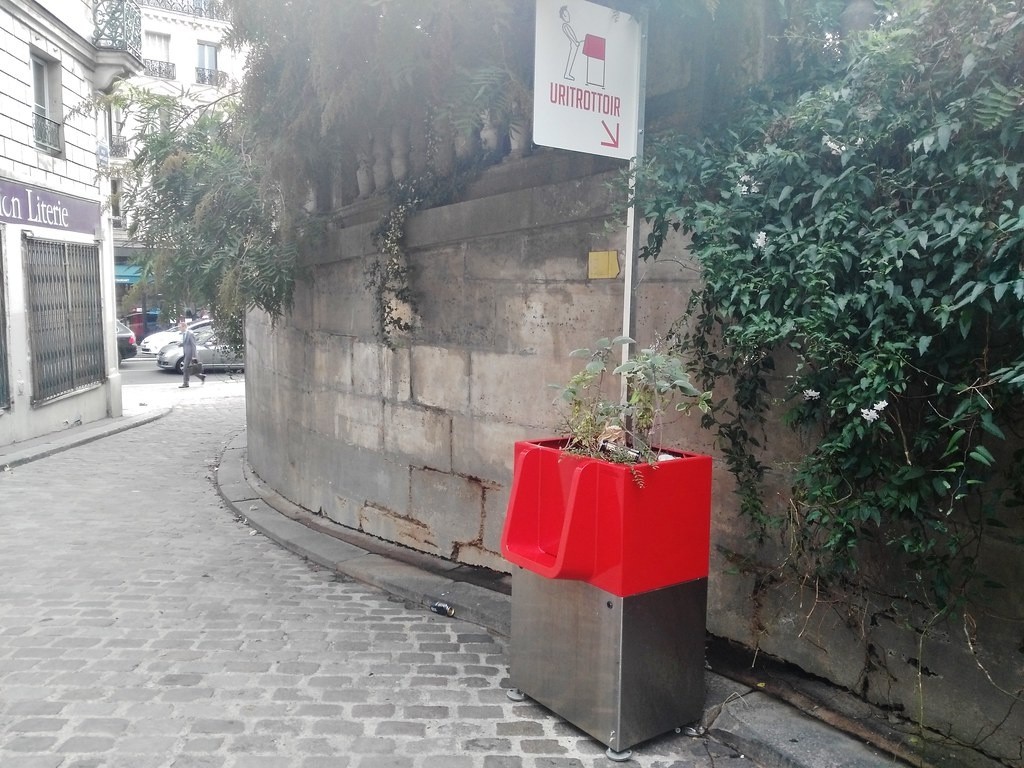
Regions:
[{"x1": 599, "y1": 439, "x2": 641, "y2": 463}]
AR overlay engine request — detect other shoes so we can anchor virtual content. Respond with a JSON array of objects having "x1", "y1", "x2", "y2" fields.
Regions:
[
  {"x1": 202, "y1": 375, "x2": 206, "y2": 383},
  {"x1": 178, "y1": 384, "x2": 189, "y2": 388}
]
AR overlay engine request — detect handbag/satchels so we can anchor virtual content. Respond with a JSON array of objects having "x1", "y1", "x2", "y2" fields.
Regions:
[{"x1": 188, "y1": 362, "x2": 203, "y2": 375}]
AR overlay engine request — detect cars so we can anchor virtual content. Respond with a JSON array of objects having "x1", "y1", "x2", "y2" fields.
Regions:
[
  {"x1": 140, "y1": 313, "x2": 220, "y2": 356},
  {"x1": 116, "y1": 319, "x2": 138, "y2": 367},
  {"x1": 155, "y1": 324, "x2": 245, "y2": 375},
  {"x1": 119, "y1": 311, "x2": 178, "y2": 346}
]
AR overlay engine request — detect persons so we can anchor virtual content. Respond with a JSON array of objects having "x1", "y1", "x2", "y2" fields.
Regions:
[{"x1": 176, "y1": 322, "x2": 207, "y2": 388}]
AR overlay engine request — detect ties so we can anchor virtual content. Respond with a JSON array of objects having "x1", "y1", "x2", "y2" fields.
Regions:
[{"x1": 183, "y1": 333, "x2": 186, "y2": 343}]
